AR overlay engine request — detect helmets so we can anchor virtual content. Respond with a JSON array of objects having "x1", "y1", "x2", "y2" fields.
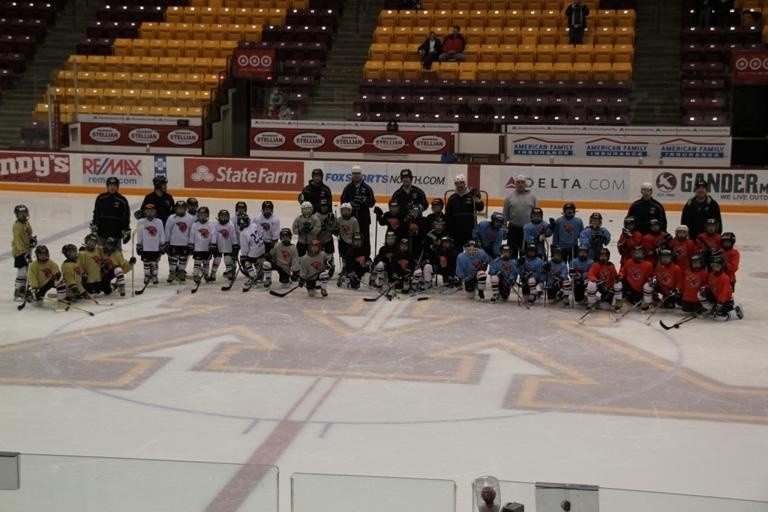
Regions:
[
  {"x1": 153, "y1": 175, "x2": 168, "y2": 188},
  {"x1": 187, "y1": 197, "x2": 199, "y2": 207},
  {"x1": 639, "y1": 183, "x2": 654, "y2": 196},
  {"x1": 174, "y1": 200, "x2": 187, "y2": 211},
  {"x1": 197, "y1": 207, "x2": 209, "y2": 217},
  {"x1": 262, "y1": 200, "x2": 273, "y2": 210},
  {"x1": 453, "y1": 173, "x2": 466, "y2": 189},
  {"x1": 35, "y1": 245, "x2": 50, "y2": 262},
  {"x1": 103, "y1": 234, "x2": 119, "y2": 248},
  {"x1": 217, "y1": 210, "x2": 230, "y2": 218},
  {"x1": 312, "y1": 168, "x2": 324, "y2": 186},
  {"x1": 400, "y1": 168, "x2": 413, "y2": 182},
  {"x1": 84, "y1": 234, "x2": 98, "y2": 245},
  {"x1": 106, "y1": 177, "x2": 120, "y2": 189},
  {"x1": 237, "y1": 214, "x2": 250, "y2": 227},
  {"x1": 142, "y1": 200, "x2": 158, "y2": 217},
  {"x1": 236, "y1": 202, "x2": 247, "y2": 213},
  {"x1": 515, "y1": 175, "x2": 527, "y2": 190},
  {"x1": 622, "y1": 217, "x2": 736, "y2": 271},
  {"x1": 351, "y1": 167, "x2": 362, "y2": 178},
  {"x1": 62, "y1": 244, "x2": 78, "y2": 261},
  {"x1": 14, "y1": 204, "x2": 31, "y2": 221},
  {"x1": 279, "y1": 201, "x2": 610, "y2": 274},
  {"x1": 692, "y1": 181, "x2": 707, "y2": 195}
]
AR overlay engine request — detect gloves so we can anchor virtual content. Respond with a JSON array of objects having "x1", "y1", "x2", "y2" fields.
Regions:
[
  {"x1": 159, "y1": 244, "x2": 166, "y2": 254},
  {"x1": 291, "y1": 271, "x2": 305, "y2": 288},
  {"x1": 137, "y1": 244, "x2": 143, "y2": 255},
  {"x1": 166, "y1": 243, "x2": 171, "y2": 254},
  {"x1": 188, "y1": 244, "x2": 194, "y2": 254},
  {"x1": 30, "y1": 238, "x2": 37, "y2": 247},
  {"x1": 122, "y1": 231, "x2": 131, "y2": 244},
  {"x1": 241, "y1": 257, "x2": 246, "y2": 265},
  {"x1": 71, "y1": 285, "x2": 79, "y2": 293},
  {"x1": 92, "y1": 228, "x2": 99, "y2": 237},
  {"x1": 210, "y1": 246, "x2": 217, "y2": 254},
  {"x1": 595, "y1": 276, "x2": 728, "y2": 316},
  {"x1": 134, "y1": 209, "x2": 143, "y2": 220},
  {"x1": 25, "y1": 253, "x2": 32, "y2": 264},
  {"x1": 129, "y1": 256, "x2": 137, "y2": 265},
  {"x1": 83, "y1": 273, "x2": 89, "y2": 282}
]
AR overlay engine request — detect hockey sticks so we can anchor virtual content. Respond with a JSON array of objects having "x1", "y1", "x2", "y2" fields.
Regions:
[
  {"x1": 562, "y1": 224, "x2": 574, "y2": 307},
  {"x1": 578, "y1": 294, "x2": 611, "y2": 324},
  {"x1": 131, "y1": 228, "x2": 151, "y2": 297},
  {"x1": 355, "y1": 250, "x2": 462, "y2": 302},
  {"x1": 267, "y1": 260, "x2": 321, "y2": 297},
  {"x1": 543, "y1": 237, "x2": 549, "y2": 306},
  {"x1": 221, "y1": 258, "x2": 262, "y2": 293},
  {"x1": 17, "y1": 247, "x2": 31, "y2": 310},
  {"x1": 39, "y1": 293, "x2": 113, "y2": 315},
  {"x1": 608, "y1": 298, "x2": 711, "y2": 329},
  {"x1": 176, "y1": 254, "x2": 211, "y2": 293},
  {"x1": 503, "y1": 275, "x2": 528, "y2": 310}
]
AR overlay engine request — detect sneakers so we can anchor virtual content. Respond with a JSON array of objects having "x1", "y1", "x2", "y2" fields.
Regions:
[
  {"x1": 204, "y1": 274, "x2": 210, "y2": 282},
  {"x1": 167, "y1": 274, "x2": 175, "y2": 283},
  {"x1": 735, "y1": 306, "x2": 743, "y2": 319},
  {"x1": 57, "y1": 299, "x2": 71, "y2": 307},
  {"x1": 144, "y1": 275, "x2": 150, "y2": 284},
  {"x1": 193, "y1": 275, "x2": 200, "y2": 282},
  {"x1": 211, "y1": 273, "x2": 216, "y2": 281},
  {"x1": 15, "y1": 288, "x2": 25, "y2": 297},
  {"x1": 263, "y1": 277, "x2": 272, "y2": 287},
  {"x1": 176, "y1": 273, "x2": 186, "y2": 282},
  {"x1": 245, "y1": 278, "x2": 257, "y2": 285},
  {"x1": 320, "y1": 278, "x2": 594, "y2": 310},
  {"x1": 152, "y1": 276, "x2": 158, "y2": 284},
  {"x1": 223, "y1": 270, "x2": 232, "y2": 276}
]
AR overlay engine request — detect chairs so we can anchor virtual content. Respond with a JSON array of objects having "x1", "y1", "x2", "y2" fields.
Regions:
[
  {"x1": 683, "y1": 2, "x2": 768, "y2": 127},
  {"x1": 19, "y1": 0, "x2": 334, "y2": 128},
  {"x1": 355, "y1": 0, "x2": 637, "y2": 127},
  {"x1": 1, "y1": 0, "x2": 53, "y2": 84}
]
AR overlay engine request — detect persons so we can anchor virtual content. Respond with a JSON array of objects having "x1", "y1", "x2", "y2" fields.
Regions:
[
  {"x1": 417, "y1": 31, "x2": 443, "y2": 70},
  {"x1": 264, "y1": 86, "x2": 284, "y2": 113},
  {"x1": 565, "y1": 0, "x2": 589, "y2": 48},
  {"x1": 278, "y1": 105, "x2": 292, "y2": 120},
  {"x1": 438, "y1": 25, "x2": 466, "y2": 62}
]
